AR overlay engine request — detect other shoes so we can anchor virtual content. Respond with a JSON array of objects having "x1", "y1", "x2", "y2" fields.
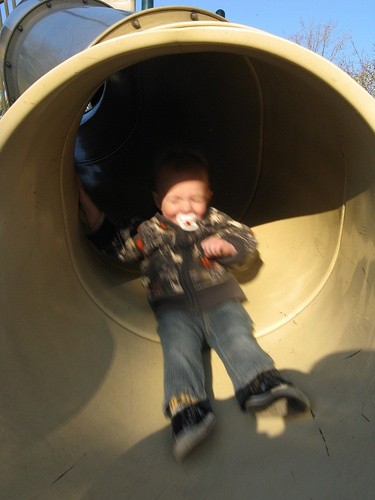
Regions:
[
  {"x1": 171, "y1": 403, "x2": 218, "y2": 464},
  {"x1": 246, "y1": 374, "x2": 310, "y2": 419}
]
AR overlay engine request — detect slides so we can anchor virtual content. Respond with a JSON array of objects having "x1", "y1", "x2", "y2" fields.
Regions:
[{"x1": 0, "y1": 0, "x2": 374, "y2": 500}]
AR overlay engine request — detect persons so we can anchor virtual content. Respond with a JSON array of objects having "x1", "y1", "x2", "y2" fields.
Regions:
[
  {"x1": 112, "y1": 152, "x2": 310, "y2": 461},
  {"x1": 78, "y1": 177, "x2": 150, "y2": 287}
]
